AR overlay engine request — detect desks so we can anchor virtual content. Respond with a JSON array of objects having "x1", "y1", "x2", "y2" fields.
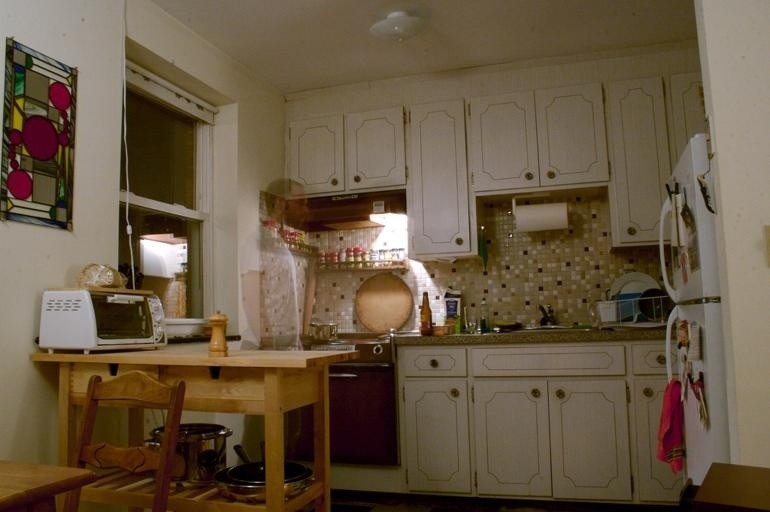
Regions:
[
  {"x1": 0, "y1": 459, "x2": 94, "y2": 511},
  {"x1": 28, "y1": 351, "x2": 362, "y2": 512}
]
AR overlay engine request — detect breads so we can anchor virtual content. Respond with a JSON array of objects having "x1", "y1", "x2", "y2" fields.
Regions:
[{"x1": 79, "y1": 265, "x2": 120, "y2": 289}]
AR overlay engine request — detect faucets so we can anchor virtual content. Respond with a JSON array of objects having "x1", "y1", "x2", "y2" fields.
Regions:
[{"x1": 538, "y1": 302, "x2": 555, "y2": 325}]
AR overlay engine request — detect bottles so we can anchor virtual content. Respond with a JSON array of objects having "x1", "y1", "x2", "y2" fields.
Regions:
[
  {"x1": 418, "y1": 290, "x2": 433, "y2": 335},
  {"x1": 209, "y1": 310, "x2": 229, "y2": 356},
  {"x1": 479, "y1": 299, "x2": 489, "y2": 334}
]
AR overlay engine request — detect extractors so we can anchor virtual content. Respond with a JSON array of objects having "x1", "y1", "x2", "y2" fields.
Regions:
[{"x1": 283, "y1": 191, "x2": 407, "y2": 232}]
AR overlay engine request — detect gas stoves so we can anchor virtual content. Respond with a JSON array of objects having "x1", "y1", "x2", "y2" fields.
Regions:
[{"x1": 300, "y1": 335, "x2": 388, "y2": 346}]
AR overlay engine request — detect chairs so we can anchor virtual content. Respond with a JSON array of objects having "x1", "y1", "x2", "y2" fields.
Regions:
[{"x1": 63, "y1": 371, "x2": 185, "y2": 511}]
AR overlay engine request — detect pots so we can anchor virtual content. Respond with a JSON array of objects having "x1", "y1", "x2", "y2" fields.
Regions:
[
  {"x1": 306, "y1": 321, "x2": 340, "y2": 343},
  {"x1": 144, "y1": 421, "x2": 233, "y2": 487},
  {"x1": 210, "y1": 442, "x2": 315, "y2": 501}
]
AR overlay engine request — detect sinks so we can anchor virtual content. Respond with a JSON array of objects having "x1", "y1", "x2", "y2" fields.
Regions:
[{"x1": 525, "y1": 325, "x2": 591, "y2": 331}]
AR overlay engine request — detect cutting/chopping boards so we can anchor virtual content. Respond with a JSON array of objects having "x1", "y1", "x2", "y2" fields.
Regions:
[{"x1": 354, "y1": 273, "x2": 415, "y2": 335}]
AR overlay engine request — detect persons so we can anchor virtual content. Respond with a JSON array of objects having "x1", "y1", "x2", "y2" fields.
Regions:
[
  {"x1": 235, "y1": 178, "x2": 318, "y2": 467},
  {"x1": 676, "y1": 323, "x2": 692, "y2": 353},
  {"x1": 682, "y1": 208, "x2": 698, "y2": 235}
]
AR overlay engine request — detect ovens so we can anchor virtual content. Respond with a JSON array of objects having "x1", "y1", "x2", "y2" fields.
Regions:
[{"x1": 284, "y1": 364, "x2": 400, "y2": 467}]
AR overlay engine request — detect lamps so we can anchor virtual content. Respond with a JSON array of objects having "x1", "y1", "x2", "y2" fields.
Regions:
[{"x1": 370, "y1": 11, "x2": 428, "y2": 43}]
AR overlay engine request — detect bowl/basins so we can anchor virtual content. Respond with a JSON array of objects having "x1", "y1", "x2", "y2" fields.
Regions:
[{"x1": 165, "y1": 318, "x2": 207, "y2": 339}]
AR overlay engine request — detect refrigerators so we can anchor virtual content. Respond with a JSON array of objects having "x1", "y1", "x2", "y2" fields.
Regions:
[{"x1": 657, "y1": 133, "x2": 730, "y2": 490}]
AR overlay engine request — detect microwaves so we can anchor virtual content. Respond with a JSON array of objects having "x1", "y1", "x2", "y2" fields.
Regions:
[{"x1": 36, "y1": 288, "x2": 168, "y2": 355}]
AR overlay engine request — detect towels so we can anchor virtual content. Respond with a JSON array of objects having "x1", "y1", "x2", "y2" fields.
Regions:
[{"x1": 655, "y1": 378, "x2": 686, "y2": 473}]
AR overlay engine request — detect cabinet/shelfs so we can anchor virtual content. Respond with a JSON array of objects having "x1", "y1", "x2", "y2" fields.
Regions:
[
  {"x1": 406, "y1": 99, "x2": 483, "y2": 263},
  {"x1": 470, "y1": 341, "x2": 630, "y2": 506},
  {"x1": 465, "y1": 82, "x2": 608, "y2": 203},
  {"x1": 397, "y1": 345, "x2": 468, "y2": 497},
  {"x1": 285, "y1": 102, "x2": 406, "y2": 198},
  {"x1": 629, "y1": 340, "x2": 687, "y2": 505},
  {"x1": 604, "y1": 70, "x2": 708, "y2": 254}
]
